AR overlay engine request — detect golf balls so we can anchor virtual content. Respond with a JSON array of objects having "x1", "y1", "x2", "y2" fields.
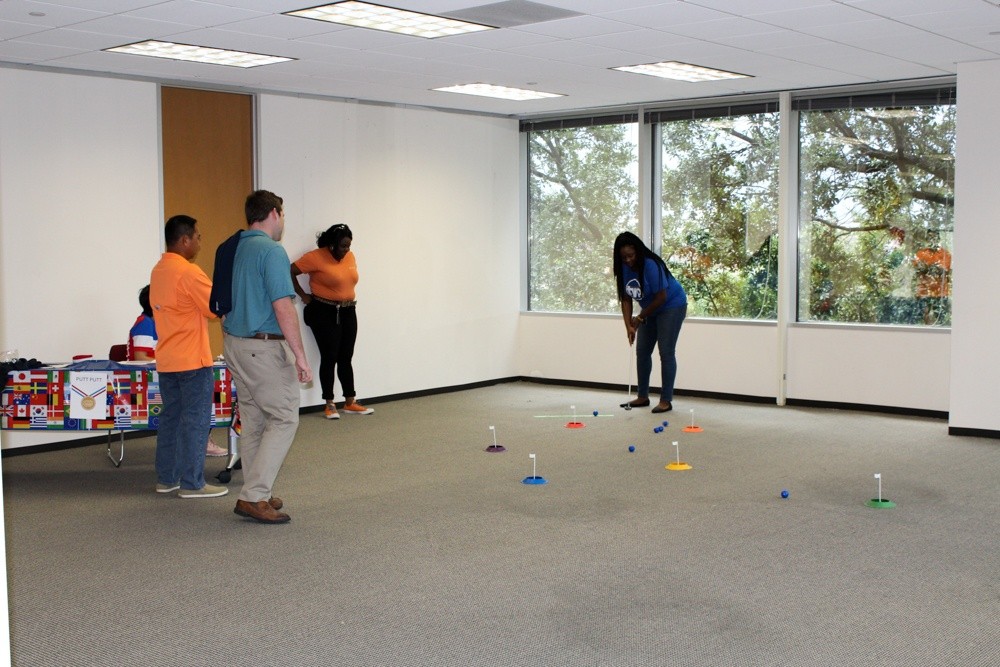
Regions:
[
  {"x1": 629, "y1": 446, "x2": 635, "y2": 452},
  {"x1": 659, "y1": 426, "x2": 664, "y2": 431},
  {"x1": 593, "y1": 410, "x2": 598, "y2": 416},
  {"x1": 654, "y1": 427, "x2": 659, "y2": 433},
  {"x1": 781, "y1": 491, "x2": 788, "y2": 498},
  {"x1": 662, "y1": 421, "x2": 669, "y2": 427}
]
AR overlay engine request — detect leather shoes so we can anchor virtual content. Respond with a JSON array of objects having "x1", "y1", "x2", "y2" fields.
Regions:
[
  {"x1": 268, "y1": 495, "x2": 283, "y2": 510},
  {"x1": 233, "y1": 498, "x2": 291, "y2": 523}
]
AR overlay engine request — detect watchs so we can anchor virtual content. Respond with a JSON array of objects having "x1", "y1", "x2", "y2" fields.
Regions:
[{"x1": 638, "y1": 315, "x2": 644, "y2": 322}]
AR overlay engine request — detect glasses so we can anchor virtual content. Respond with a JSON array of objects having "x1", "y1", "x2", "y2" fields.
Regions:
[{"x1": 332, "y1": 224, "x2": 349, "y2": 232}]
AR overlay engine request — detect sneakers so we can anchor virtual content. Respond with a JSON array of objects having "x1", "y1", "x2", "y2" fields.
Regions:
[
  {"x1": 325, "y1": 404, "x2": 340, "y2": 419},
  {"x1": 343, "y1": 402, "x2": 375, "y2": 415},
  {"x1": 205, "y1": 436, "x2": 229, "y2": 457}
]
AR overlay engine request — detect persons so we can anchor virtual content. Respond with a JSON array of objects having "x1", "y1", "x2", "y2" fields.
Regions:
[
  {"x1": 290, "y1": 224, "x2": 374, "y2": 418},
  {"x1": 127, "y1": 189, "x2": 312, "y2": 523},
  {"x1": 613, "y1": 231, "x2": 687, "y2": 413}
]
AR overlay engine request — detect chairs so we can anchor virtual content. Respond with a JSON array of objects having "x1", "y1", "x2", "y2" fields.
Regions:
[{"x1": 107, "y1": 344, "x2": 127, "y2": 467}]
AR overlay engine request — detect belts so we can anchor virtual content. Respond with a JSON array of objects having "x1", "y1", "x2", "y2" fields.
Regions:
[
  {"x1": 226, "y1": 330, "x2": 288, "y2": 340},
  {"x1": 314, "y1": 295, "x2": 357, "y2": 307}
]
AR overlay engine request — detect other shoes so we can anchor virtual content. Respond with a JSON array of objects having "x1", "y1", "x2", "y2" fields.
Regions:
[
  {"x1": 178, "y1": 484, "x2": 229, "y2": 499},
  {"x1": 651, "y1": 402, "x2": 672, "y2": 413},
  {"x1": 156, "y1": 482, "x2": 181, "y2": 493},
  {"x1": 619, "y1": 398, "x2": 650, "y2": 407}
]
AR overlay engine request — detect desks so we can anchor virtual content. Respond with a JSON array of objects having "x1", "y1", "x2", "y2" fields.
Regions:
[{"x1": 0, "y1": 360, "x2": 242, "y2": 484}]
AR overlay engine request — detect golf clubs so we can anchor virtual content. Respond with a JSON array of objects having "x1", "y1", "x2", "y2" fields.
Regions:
[{"x1": 625, "y1": 327, "x2": 634, "y2": 410}]
